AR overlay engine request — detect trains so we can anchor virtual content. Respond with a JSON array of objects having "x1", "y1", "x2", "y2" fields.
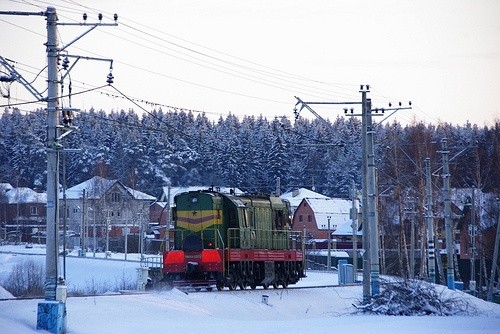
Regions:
[{"x1": 163, "y1": 189, "x2": 305, "y2": 292}]
